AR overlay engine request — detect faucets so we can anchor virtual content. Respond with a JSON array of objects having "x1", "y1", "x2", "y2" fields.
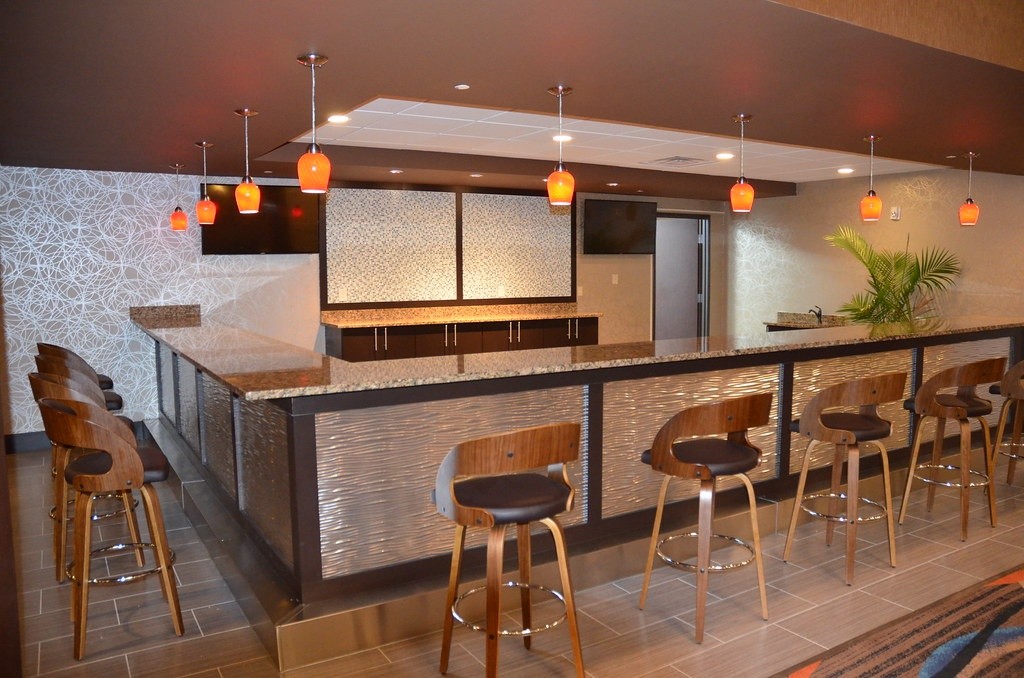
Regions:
[{"x1": 808, "y1": 306, "x2": 822, "y2": 323}]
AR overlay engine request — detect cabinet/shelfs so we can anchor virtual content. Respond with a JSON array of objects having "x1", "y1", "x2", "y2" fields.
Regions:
[{"x1": 339, "y1": 318, "x2": 597, "y2": 364}]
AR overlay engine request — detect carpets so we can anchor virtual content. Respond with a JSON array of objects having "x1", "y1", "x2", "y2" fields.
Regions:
[{"x1": 768, "y1": 563, "x2": 1024, "y2": 678}]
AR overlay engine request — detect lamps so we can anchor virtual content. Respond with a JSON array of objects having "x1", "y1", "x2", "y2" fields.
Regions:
[
  {"x1": 548, "y1": 85, "x2": 574, "y2": 206},
  {"x1": 234, "y1": 108, "x2": 260, "y2": 213},
  {"x1": 296, "y1": 54, "x2": 331, "y2": 193},
  {"x1": 194, "y1": 141, "x2": 216, "y2": 225},
  {"x1": 860, "y1": 133, "x2": 883, "y2": 222},
  {"x1": 169, "y1": 161, "x2": 187, "y2": 231},
  {"x1": 731, "y1": 112, "x2": 753, "y2": 212},
  {"x1": 960, "y1": 151, "x2": 980, "y2": 225}
]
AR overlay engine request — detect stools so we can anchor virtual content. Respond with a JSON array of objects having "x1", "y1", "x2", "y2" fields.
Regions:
[
  {"x1": 783, "y1": 372, "x2": 909, "y2": 588},
  {"x1": 983, "y1": 359, "x2": 1024, "y2": 494},
  {"x1": 429, "y1": 421, "x2": 584, "y2": 678},
  {"x1": 898, "y1": 356, "x2": 1007, "y2": 541},
  {"x1": 638, "y1": 393, "x2": 774, "y2": 644},
  {"x1": 28, "y1": 342, "x2": 185, "y2": 661}
]
